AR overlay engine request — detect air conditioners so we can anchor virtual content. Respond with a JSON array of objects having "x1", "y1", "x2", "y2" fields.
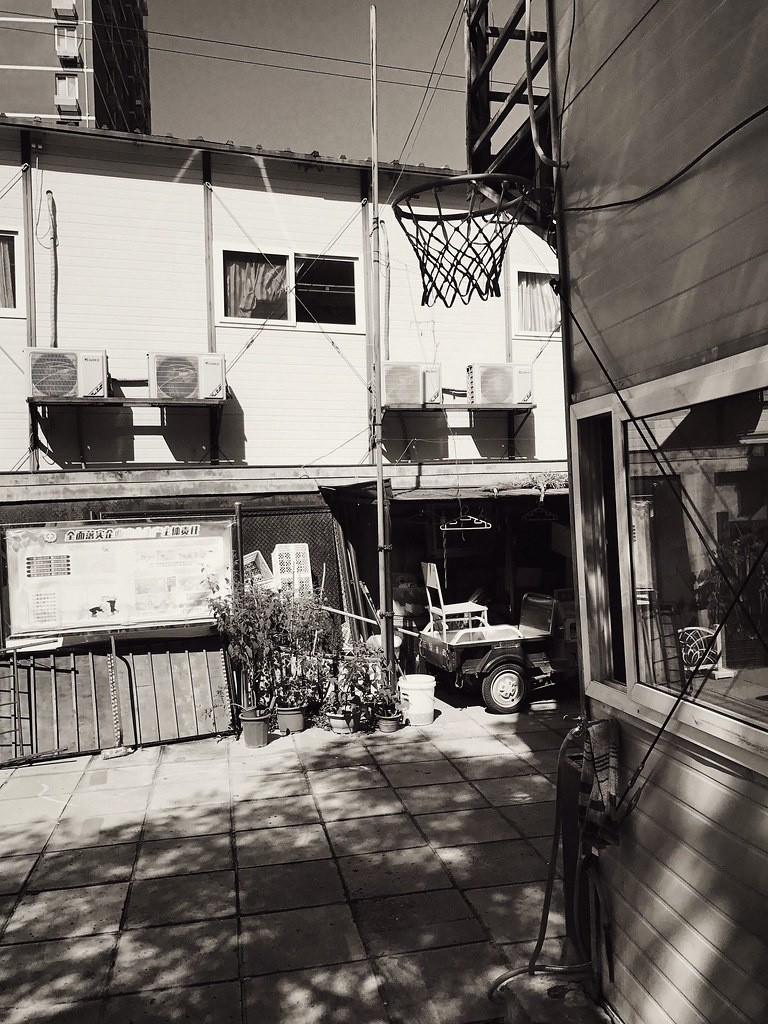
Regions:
[
  {"x1": 25, "y1": 349, "x2": 108, "y2": 398},
  {"x1": 469, "y1": 364, "x2": 532, "y2": 406},
  {"x1": 379, "y1": 362, "x2": 443, "y2": 409},
  {"x1": 147, "y1": 352, "x2": 228, "y2": 401}
]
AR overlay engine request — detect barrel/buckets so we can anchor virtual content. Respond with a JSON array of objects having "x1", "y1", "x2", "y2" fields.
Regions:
[
  {"x1": 366, "y1": 634, "x2": 403, "y2": 671},
  {"x1": 396, "y1": 674, "x2": 437, "y2": 725}
]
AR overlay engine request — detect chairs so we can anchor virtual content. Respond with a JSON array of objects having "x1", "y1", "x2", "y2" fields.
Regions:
[{"x1": 421, "y1": 560, "x2": 488, "y2": 640}]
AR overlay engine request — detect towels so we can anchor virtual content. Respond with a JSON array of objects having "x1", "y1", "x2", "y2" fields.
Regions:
[{"x1": 581, "y1": 717, "x2": 618, "y2": 813}]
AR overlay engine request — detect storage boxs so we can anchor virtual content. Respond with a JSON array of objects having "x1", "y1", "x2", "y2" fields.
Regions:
[{"x1": 244, "y1": 550, "x2": 276, "y2": 593}]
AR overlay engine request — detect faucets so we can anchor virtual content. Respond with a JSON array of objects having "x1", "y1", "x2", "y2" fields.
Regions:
[{"x1": 563, "y1": 713, "x2": 587, "y2": 741}]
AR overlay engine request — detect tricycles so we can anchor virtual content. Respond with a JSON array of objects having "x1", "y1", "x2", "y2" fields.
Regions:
[{"x1": 416, "y1": 588, "x2": 574, "y2": 715}]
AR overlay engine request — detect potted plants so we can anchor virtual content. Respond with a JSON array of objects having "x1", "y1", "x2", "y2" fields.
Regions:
[{"x1": 200, "y1": 576, "x2": 408, "y2": 746}]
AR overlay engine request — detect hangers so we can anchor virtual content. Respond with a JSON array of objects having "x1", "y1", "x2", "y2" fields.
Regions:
[{"x1": 439, "y1": 505, "x2": 558, "y2": 530}]
